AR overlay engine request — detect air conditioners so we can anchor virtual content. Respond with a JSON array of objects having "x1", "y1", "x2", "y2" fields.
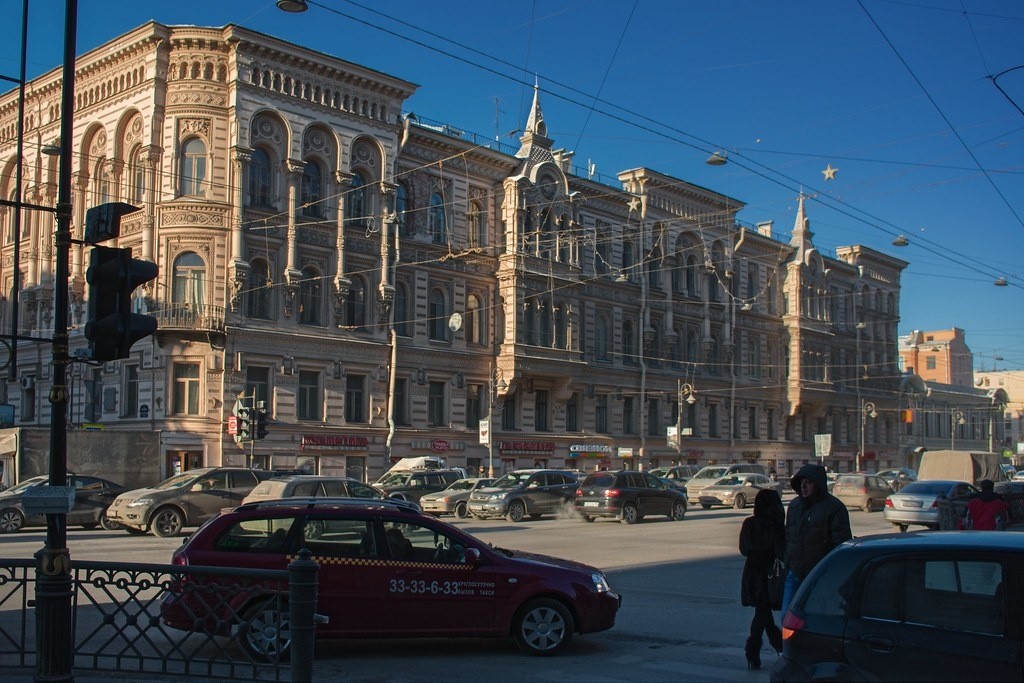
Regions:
[{"x1": 20, "y1": 377, "x2": 34, "y2": 390}]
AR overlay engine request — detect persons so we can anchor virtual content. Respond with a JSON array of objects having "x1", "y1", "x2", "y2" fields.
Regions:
[
  {"x1": 780, "y1": 464, "x2": 853, "y2": 623},
  {"x1": 958, "y1": 479, "x2": 1008, "y2": 531},
  {"x1": 739, "y1": 489, "x2": 787, "y2": 670},
  {"x1": 478, "y1": 466, "x2": 487, "y2": 488}
]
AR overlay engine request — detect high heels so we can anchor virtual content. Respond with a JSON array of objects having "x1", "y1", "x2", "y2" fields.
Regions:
[
  {"x1": 744, "y1": 636, "x2": 762, "y2": 670},
  {"x1": 766, "y1": 625, "x2": 783, "y2": 656}
]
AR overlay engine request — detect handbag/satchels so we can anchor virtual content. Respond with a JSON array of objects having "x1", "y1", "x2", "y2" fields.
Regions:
[{"x1": 767, "y1": 557, "x2": 785, "y2": 611}]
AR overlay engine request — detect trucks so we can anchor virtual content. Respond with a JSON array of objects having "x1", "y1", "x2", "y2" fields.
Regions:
[{"x1": 917, "y1": 450, "x2": 1010, "y2": 489}]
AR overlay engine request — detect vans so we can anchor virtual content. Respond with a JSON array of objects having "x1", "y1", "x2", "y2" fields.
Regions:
[{"x1": 684, "y1": 464, "x2": 764, "y2": 506}]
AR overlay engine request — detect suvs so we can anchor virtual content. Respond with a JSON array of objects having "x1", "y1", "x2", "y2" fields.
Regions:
[
  {"x1": 466, "y1": 469, "x2": 581, "y2": 522},
  {"x1": 648, "y1": 465, "x2": 701, "y2": 488},
  {"x1": 575, "y1": 470, "x2": 688, "y2": 525},
  {"x1": 370, "y1": 467, "x2": 466, "y2": 504}
]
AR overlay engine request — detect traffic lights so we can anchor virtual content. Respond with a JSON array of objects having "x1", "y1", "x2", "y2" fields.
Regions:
[
  {"x1": 238, "y1": 407, "x2": 253, "y2": 440},
  {"x1": 256, "y1": 410, "x2": 269, "y2": 440},
  {"x1": 86, "y1": 246, "x2": 158, "y2": 364}
]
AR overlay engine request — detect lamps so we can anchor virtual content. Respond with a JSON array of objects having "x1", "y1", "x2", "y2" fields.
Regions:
[
  {"x1": 705, "y1": 151, "x2": 727, "y2": 165},
  {"x1": 892, "y1": 235, "x2": 908, "y2": 247},
  {"x1": 993, "y1": 277, "x2": 1007, "y2": 286},
  {"x1": 42, "y1": 146, "x2": 61, "y2": 157},
  {"x1": 276, "y1": 0, "x2": 308, "y2": 13}
]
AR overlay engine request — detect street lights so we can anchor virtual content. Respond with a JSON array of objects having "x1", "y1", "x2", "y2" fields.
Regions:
[
  {"x1": 676, "y1": 384, "x2": 697, "y2": 464},
  {"x1": 861, "y1": 402, "x2": 877, "y2": 469},
  {"x1": 489, "y1": 367, "x2": 510, "y2": 477},
  {"x1": 951, "y1": 411, "x2": 969, "y2": 452}
]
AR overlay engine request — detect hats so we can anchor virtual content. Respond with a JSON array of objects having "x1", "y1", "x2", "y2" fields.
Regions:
[{"x1": 479, "y1": 466, "x2": 485, "y2": 469}]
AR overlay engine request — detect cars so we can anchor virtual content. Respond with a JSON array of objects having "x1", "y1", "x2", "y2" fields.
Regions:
[
  {"x1": 1011, "y1": 470, "x2": 1024, "y2": 482},
  {"x1": 883, "y1": 479, "x2": 980, "y2": 533},
  {"x1": 769, "y1": 528, "x2": 1023, "y2": 683},
  {"x1": 418, "y1": 477, "x2": 497, "y2": 519},
  {"x1": 698, "y1": 473, "x2": 783, "y2": 508},
  {"x1": 0, "y1": 472, "x2": 129, "y2": 534},
  {"x1": 239, "y1": 475, "x2": 422, "y2": 538},
  {"x1": 1001, "y1": 464, "x2": 1017, "y2": 476},
  {"x1": 105, "y1": 466, "x2": 291, "y2": 538},
  {"x1": 827, "y1": 472, "x2": 841, "y2": 492},
  {"x1": 832, "y1": 475, "x2": 894, "y2": 513},
  {"x1": 159, "y1": 499, "x2": 623, "y2": 662},
  {"x1": 874, "y1": 468, "x2": 917, "y2": 492}
]
